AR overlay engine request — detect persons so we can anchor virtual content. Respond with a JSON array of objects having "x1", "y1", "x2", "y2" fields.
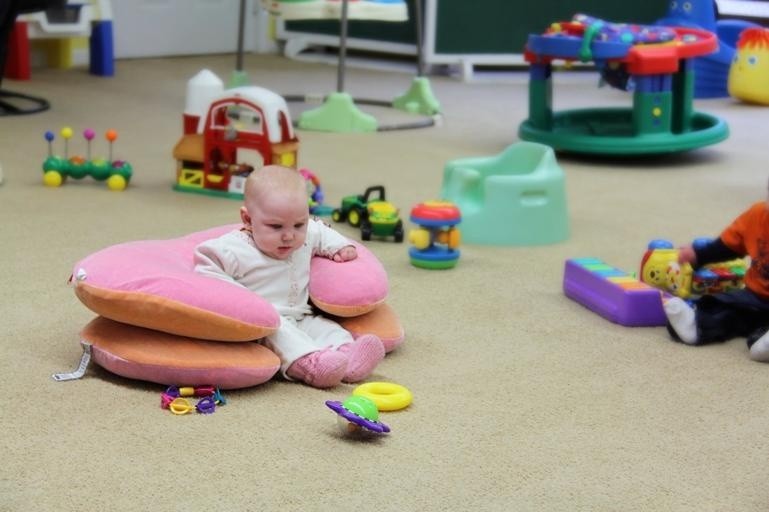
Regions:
[
  {"x1": 198, "y1": 162, "x2": 385, "y2": 387},
  {"x1": 663, "y1": 200, "x2": 769, "y2": 363}
]
